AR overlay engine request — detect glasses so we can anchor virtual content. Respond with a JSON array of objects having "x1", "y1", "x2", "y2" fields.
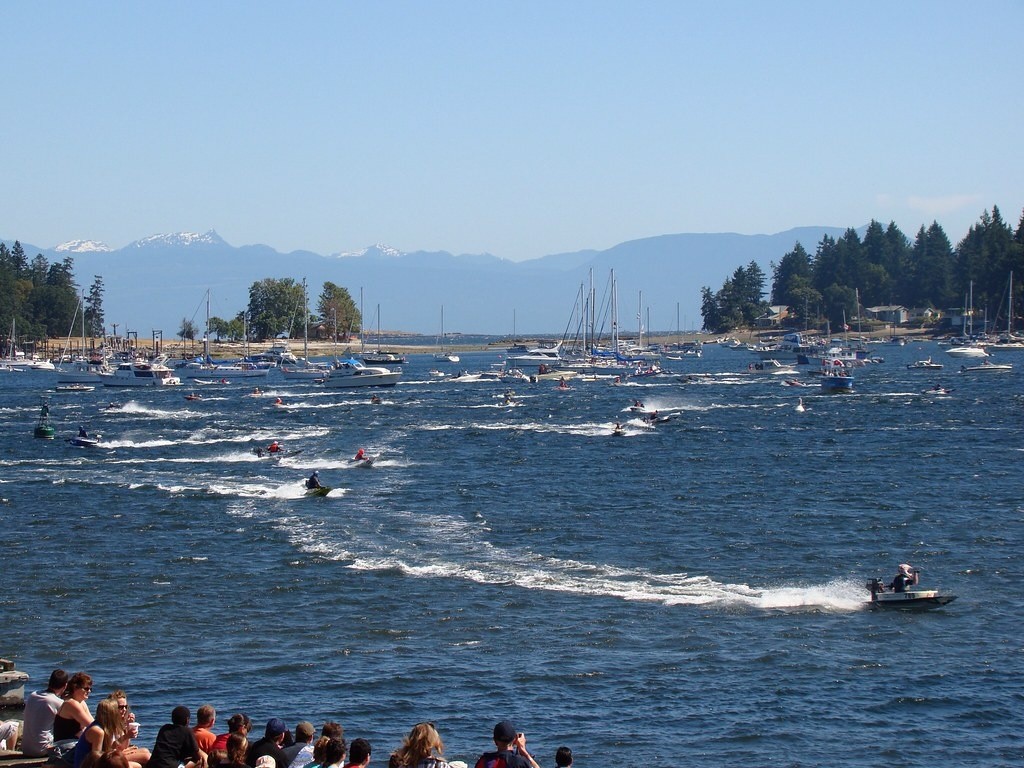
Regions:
[
  {"x1": 415, "y1": 722, "x2": 434, "y2": 730},
  {"x1": 80, "y1": 686, "x2": 92, "y2": 692},
  {"x1": 116, "y1": 704, "x2": 128, "y2": 710}
]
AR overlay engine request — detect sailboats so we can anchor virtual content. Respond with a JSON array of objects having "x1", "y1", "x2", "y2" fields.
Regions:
[
  {"x1": 946, "y1": 280, "x2": 990, "y2": 358},
  {"x1": 963, "y1": 271, "x2": 1024, "y2": 352},
  {"x1": 496, "y1": 268, "x2": 702, "y2": 374},
  {"x1": 797, "y1": 288, "x2": 883, "y2": 366},
  {"x1": 1, "y1": 275, "x2": 460, "y2": 400}
]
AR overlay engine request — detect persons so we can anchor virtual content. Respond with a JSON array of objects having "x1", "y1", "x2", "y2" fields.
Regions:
[
  {"x1": 0, "y1": 669, "x2": 573, "y2": 768},
  {"x1": 748, "y1": 334, "x2": 996, "y2": 392},
  {"x1": 890, "y1": 562, "x2": 919, "y2": 593},
  {"x1": 37, "y1": 357, "x2": 667, "y2": 491}
]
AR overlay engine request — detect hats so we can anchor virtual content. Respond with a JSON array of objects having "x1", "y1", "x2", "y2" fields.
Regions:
[
  {"x1": 255, "y1": 755, "x2": 276, "y2": 768},
  {"x1": 266, "y1": 718, "x2": 288, "y2": 736},
  {"x1": 493, "y1": 722, "x2": 516, "y2": 743},
  {"x1": 295, "y1": 721, "x2": 316, "y2": 739}
]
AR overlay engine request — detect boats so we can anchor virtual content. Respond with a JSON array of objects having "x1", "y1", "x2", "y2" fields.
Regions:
[
  {"x1": 786, "y1": 380, "x2": 822, "y2": 387},
  {"x1": 865, "y1": 577, "x2": 960, "y2": 612},
  {"x1": 252, "y1": 445, "x2": 380, "y2": 497},
  {"x1": 808, "y1": 370, "x2": 839, "y2": 376},
  {"x1": 961, "y1": 360, "x2": 1012, "y2": 373},
  {"x1": 882, "y1": 336, "x2": 904, "y2": 346},
  {"x1": 66, "y1": 434, "x2": 103, "y2": 446},
  {"x1": 721, "y1": 339, "x2": 776, "y2": 354},
  {"x1": 907, "y1": 361, "x2": 944, "y2": 369},
  {"x1": 822, "y1": 358, "x2": 855, "y2": 393},
  {"x1": 850, "y1": 336, "x2": 867, "y2": 342},
  {"x1": 749, "y1": 360, "x2": 796, "y2": 374},
  {"x1": 614, "y1": 406, "x2": 683, "y2": 435},
  {"x1": 937, "y1": 337, "x2": 972, "y2": 349},
  {"x1": 429, "y1": 369, "x2": 499, "y2": 383}
]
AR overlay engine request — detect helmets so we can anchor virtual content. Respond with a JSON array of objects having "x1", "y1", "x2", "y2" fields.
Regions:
[
  {"x1": 359, "y1": 449, "x2": 364, "y2": 454},
  {"x1": 314, "y1": 470, "x2": 319, "y2": 475},
  {"x1": 898, "y1": 563, "x2": 913, "y2": 578},
  {"x1": 273, "y1": 441, "x2": 278, "y2": 444}
]
